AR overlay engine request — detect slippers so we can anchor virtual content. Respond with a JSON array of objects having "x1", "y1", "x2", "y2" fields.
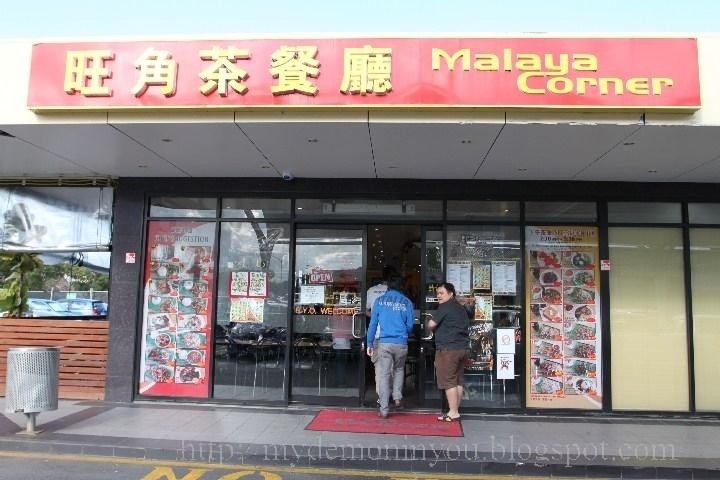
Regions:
[{"x1": 437, "y1": 414, "x2": 461, "y2": 422}]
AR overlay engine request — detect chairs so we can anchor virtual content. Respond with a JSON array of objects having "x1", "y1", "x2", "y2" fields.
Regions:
[{"x1": 221, "y1": 337, "x2": 331, "y2": 398}]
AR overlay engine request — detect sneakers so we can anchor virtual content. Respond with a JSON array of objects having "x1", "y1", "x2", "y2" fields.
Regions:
[{"x1": 377, "y1": 402, "x2": 404, "y2": 419}]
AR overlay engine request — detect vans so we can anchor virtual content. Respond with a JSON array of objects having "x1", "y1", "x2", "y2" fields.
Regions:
[{"x1": 57, "y1": 298, "x2": 104, "y2": 316}]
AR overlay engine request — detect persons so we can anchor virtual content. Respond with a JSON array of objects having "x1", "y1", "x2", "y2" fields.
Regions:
[
  {"x1": 331, "y1": 284, "x2": 354, "y2": 391},
  {"x1": 218, "y1": 298, "x2": 331, "y2": 387},
  {"x1": 363, "y1": 272, "x2": 416, "y2": 421},
  {"x1": 427, "y1": 281, "x2": 472, "y2": 424},
  {"x1": 365, "y1": 262, "x2": 400, "y2": 406}
]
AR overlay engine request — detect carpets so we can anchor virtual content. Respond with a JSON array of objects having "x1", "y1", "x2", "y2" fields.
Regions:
[{"x1": 304, "y1": 409, "x2": 464, "y2": 437}]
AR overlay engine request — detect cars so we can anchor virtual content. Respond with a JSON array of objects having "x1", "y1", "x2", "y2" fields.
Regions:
[{"x1": 26, "y1": 297, "x2": 73, "y2": 317}]
